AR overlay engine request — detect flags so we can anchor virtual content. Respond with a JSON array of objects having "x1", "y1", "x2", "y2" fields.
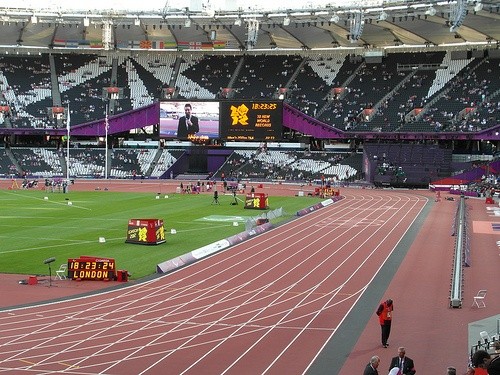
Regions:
[
  {"x1": 115, "y1": 40, "x2": 163, "y2": 49},
  {"x1": 164, "y1": 41, "x2": 226, "y2": 49},
  {"x1": 53, "y1": 39, "x2": 102, "y2": 48}
]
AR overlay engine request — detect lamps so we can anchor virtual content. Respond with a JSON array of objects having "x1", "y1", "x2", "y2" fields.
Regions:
[
  {"x1": 378, "y1": 9, "x2": 388, "y2": 22},
  {"x1": 184, "y1": 18, "x2": 192, "y2": 27},
  {"x1": 135, "y1": 18, "x2": 140, "y2": 26},
  {"x1": 283, "y1": 15, "x2": 291, "y2": 25},
  {"x1": 234, "y1": 18, "x2": 242, "y2": 26},
  {"x1": 425, "y1": 5, "x2": 436, "y2": 16},
  {"x1": 472, "y1": 2, "x2": 483, "y2": 11},
  {"x1": 31, "y1": 15, "x2": 38, "y2": 25},
  {"x1": 330, "y1": 12, "x2": 339, "y2": 24},
  {"x1": 83, "y1": 17, "x2": 89, "y2": 26}
]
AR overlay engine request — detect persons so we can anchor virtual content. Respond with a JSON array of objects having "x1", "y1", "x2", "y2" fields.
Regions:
[
  {"x1": 376, "y1": 299, "x2": 393, "y2": 348},
  {"x1": 389, "y1": 347, "x2": 416, "y2": 375},
  {"x1": 467, "y1": 350, "x2": 500, "y2": 375},
  {"x1": 0, "y1": 56, "x2": 499, "y2": 203},
  {"x1": 364, "y1": 355, "x2": 380, "y2": 375},
  {"x1": 177, "y1": 104, "x2": 199, "y2": 137}
]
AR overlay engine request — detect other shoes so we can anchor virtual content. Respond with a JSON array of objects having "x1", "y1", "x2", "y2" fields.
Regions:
[{"x1": 383, "y1": 342, "x2": 390, "y2": 348}]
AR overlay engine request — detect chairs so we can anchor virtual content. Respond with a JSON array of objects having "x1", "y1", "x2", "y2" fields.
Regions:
[
  {"x1": 54, "y1": 264, "x2": 67, "y2": 281},
  {"x1": 471, "y1": 290, "x2": 487, "y2": 309},
  {"x1": 480, "y1": 331, "x2": 494, "y2": 349}
]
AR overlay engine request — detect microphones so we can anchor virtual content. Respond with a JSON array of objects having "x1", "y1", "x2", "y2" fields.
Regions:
[
  {"x1": 44, "y1": 257, "x2": 56, "y2": 264},
  {"x1": 186, "y1": 114, "x2": 190, "y2": 120}
]
